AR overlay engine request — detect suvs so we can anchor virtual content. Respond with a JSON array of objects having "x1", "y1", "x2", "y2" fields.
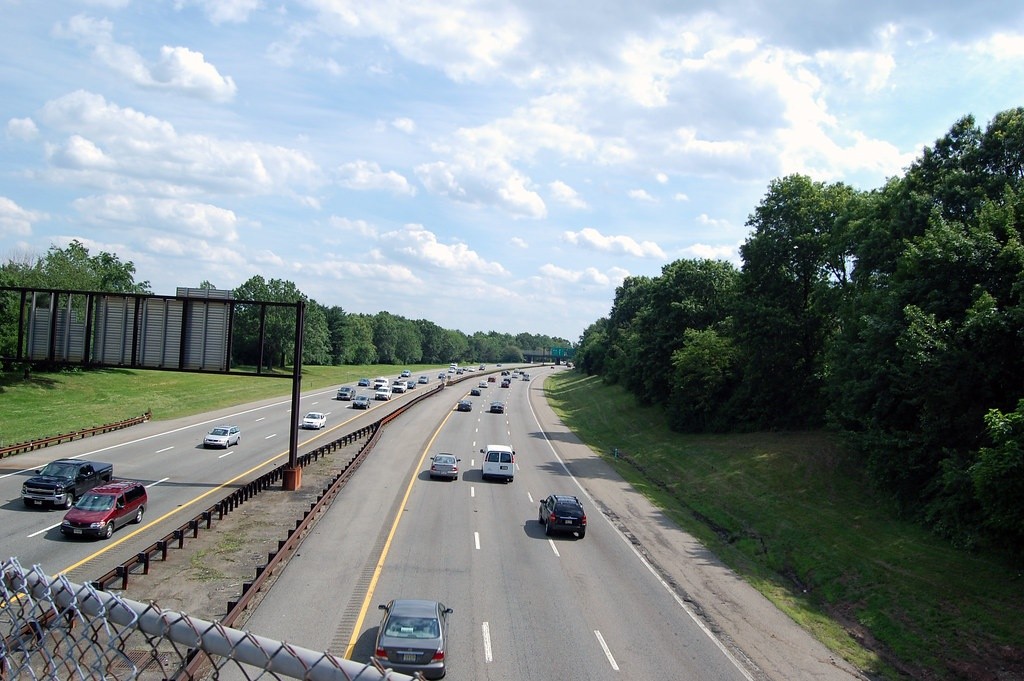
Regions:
[
  {"x1": 539, "y1": 495, "x2": 587, "y2": 539},
  {"x1": 203, "y1": 425, "x2": 242, "y2": 449},
  {"x1": 59, "y1": 479, "x2": 149, "y2": 540}
]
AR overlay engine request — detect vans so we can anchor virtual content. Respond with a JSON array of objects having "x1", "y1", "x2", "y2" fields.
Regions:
[{"x1": 480, "y1": 445, "x2": 516, "y2": 482}]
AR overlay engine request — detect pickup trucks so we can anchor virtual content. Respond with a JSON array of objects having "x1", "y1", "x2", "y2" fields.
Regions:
[{"x1": 21, "y1": 458, "x2": 113, "y2": 510}]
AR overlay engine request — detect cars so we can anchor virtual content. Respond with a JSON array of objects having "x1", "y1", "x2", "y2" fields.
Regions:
[
  {"x1": 438, "y1": 374, "x2": 446, "y2": 379},
  {"x1": 401, "y1": 370, "x2": 411, "y2": 377},
  {"x1": 373, "y1": 378, "x2": 389, "y2": 389},
  {"x1": 418, "y1": 376, "x2": 429, "y2": 384},
  {"x1": 374, "y1": 598, "x2": 453, "y2": 681},
  {"x1": 457, "y1": 400, "x2": 473, "y2": 412},
  {"x1": 479, "y1": 381, "x2": 488, "y2": 388},
  {"x1": 374, "y1": 386, "x2": 392, "y2": 401},
  {"x1": 302, "y1": 412, "x2": 327, "y2": 429},
  {"x1": 392, "y1": 379, "x2": 416, "y2": 394},
  {"x1": 337, "y1": 386, "x2": 356, "y2": 401},
  {"x1": 500, "y1": 368, "x2": 530, "y2": 383},
  {"x1": 490, "y1": 401, "x2": 505, "y2": 414},
  {"x1": 352, "y1": 395, "x2": 372, "y2": 410},
  {"x1": 471, "y1": 387, "x2": 482, "y2": 396},
  {"x1": 501, "y1": 381, "x2": 509, "y2": 388},
  {"x1": 497, "y1": 363, "x2": 501, "y2": 368},
  {"x1": 448, "y1": 363, "x2": 486, "y2": 374},
  {"x1": 429, "y1": 452, "x2": 461, "y2": 481},
  {"x1": 488, "y1": 376, "x2": 496, "y2": 382},
  {"x1": 358, "y1": 378, "x2": 371, "y2": 387}
]
